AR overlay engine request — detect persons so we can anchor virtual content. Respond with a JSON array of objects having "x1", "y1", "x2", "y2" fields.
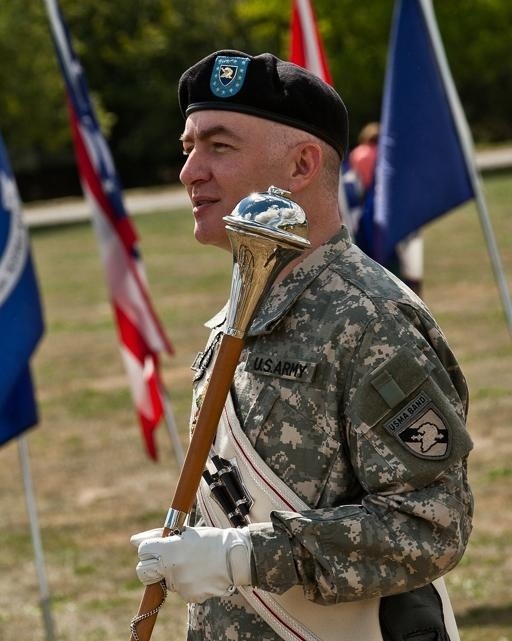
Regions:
[
  {"x1": 341, "y1": 122, "x2": 425, "y2": 298},
  {"x1": 130, "y1": 49, "x2": 473, "y2": 641}
]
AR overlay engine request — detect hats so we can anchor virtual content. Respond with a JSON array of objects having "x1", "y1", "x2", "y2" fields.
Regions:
[{"x1": 178, "y1": 49, "x2": 349, "y2": 159}]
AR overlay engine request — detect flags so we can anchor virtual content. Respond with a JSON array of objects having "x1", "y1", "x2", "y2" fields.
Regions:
[
  {"x1": 43, "y1": 1, "x2": 177, "y2": 462},
  {"x1": 277, "y1": 0, "x2": 338, "y2": 83},
  {"x1": 0, "y1": 143, "x2": 46, "y2": 446},
  {"x1": 355, "y1": 0, "x2": 479, "y2": 270}
]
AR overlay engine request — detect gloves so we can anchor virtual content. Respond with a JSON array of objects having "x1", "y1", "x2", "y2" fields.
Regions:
[{"x1": 130, "y1": 526, "x2": 257, "y2": 602}]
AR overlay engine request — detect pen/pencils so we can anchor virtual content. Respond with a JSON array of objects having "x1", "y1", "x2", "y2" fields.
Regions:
[{"x1": 203, "y1": 443, "x2": 252, "y2": 529}]
